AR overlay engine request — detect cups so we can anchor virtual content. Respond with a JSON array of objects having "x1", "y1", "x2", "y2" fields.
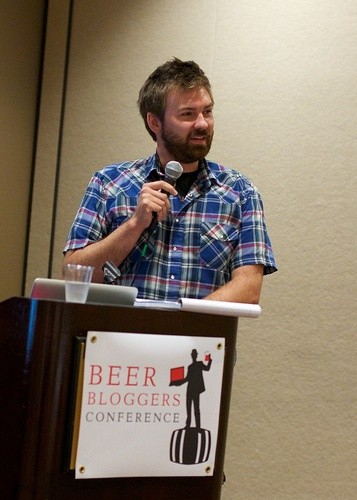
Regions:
[{"x1": 64, "y1": 263, "x2": 95, "y2": 304}]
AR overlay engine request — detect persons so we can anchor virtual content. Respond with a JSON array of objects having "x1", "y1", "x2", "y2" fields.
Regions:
[{"x1": 58, "y1": 55, "x2": 278, "y2": 305}]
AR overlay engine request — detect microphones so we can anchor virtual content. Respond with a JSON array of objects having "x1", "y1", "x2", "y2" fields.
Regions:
[{"x1": 147, "y1": 161, "x2": 184, "y2": 236}]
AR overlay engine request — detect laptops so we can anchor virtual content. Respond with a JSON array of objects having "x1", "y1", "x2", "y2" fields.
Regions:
[{"x1": 29, "y1": 277, "x2": 140, "y2": 310}]
